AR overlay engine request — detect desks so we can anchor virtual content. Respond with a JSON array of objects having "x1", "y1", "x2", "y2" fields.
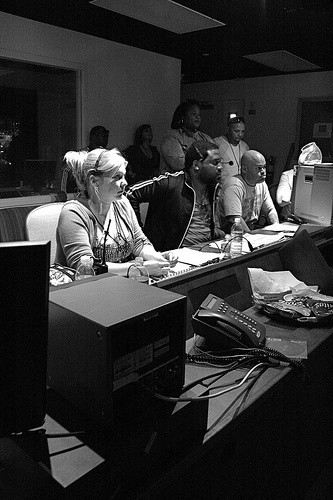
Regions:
[{"x1": 0, "y1": 222, "x2": 333, "y2": 500}]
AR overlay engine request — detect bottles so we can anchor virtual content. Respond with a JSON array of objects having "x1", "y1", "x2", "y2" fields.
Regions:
[
  {"x1": 229, "y1": 218, "x2": 242, "y2": 256},
  {"x1": 130, "y1": 257, "x2": 150, "y2": 285},
  {"x1": 74, "y1": 256, "x2": 95, "y2": 281}
]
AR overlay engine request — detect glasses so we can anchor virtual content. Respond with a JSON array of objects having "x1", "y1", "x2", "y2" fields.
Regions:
[{"x1": 233, "y1": 117, "x2": 246, "y2": 123}]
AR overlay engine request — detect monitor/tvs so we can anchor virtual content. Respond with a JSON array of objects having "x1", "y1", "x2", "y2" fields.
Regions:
[{"x1": 291, "y1": 163, "x2": 333, "y2": 227}]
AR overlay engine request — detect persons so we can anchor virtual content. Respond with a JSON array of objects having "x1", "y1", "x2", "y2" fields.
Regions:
[
  {"x1": 120, "y1": 124, "x2": 162, "y2": 186},
  {"x1": 221, "y1": 150, "x2": 281, "y2": 235},
  {"x1": 212, "y1": 116, "x2": 250, "y2": 180},
  {"x1": 121, "y1": 140, "x2": 225, "y2": 252},
  {"x1": 159, "y1": 102, "x2": 215, "y2": 174},
  {"x1": 59, "y1": 125, "x2": 118, "y2": 190},
  {"x1": 55, "y1": 147, "x2": 171, "y2": 279},
  {"x1": 274, "y1": 142, "x2": 321, "y2": 207}
]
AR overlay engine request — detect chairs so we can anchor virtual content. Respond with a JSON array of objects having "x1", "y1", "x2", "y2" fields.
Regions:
[{"x1": 26, "y1": 202, "x2": 65, "y2": 265}]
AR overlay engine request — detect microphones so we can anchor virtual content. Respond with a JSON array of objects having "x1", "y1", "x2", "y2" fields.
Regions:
[
  {"x1": 221, "y1": 161, "x2": 233, "y2": 165},
  {"x1": 95, "y1": 219, "x2": 111, "y2": 274}
]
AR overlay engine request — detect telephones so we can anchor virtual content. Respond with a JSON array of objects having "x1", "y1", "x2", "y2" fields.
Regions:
[{"x1": 192, "y1": 293, "x2": 267, "y2": 354}]
